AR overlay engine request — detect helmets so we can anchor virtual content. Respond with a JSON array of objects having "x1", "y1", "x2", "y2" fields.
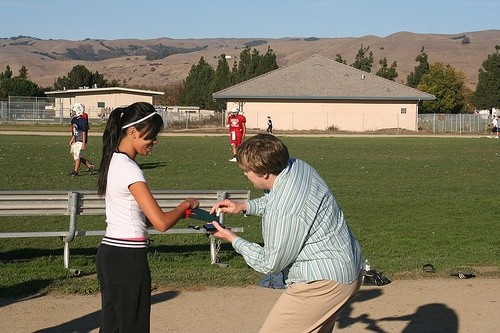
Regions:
[{"x1": 71, "y1": 103, "x2": 85, "y2": 117}]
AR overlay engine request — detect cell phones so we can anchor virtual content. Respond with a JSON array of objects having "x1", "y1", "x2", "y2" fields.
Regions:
[{"x1": 203, "y1": 224, "x2": 226, "y2": 231}]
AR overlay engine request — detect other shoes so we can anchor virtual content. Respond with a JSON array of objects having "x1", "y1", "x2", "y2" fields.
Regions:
[
  {"x1": 229, "y1": 157, "x2": 237, "y2": 162},
  {"x1": 85, "y1": 163, "x2": 95, "y2": 173},
  {"x1": 69, "y1": 171, "x2": 78, "y2": 177},
  {"x1": 72, "y1": 167, "x2": 82, "y2": 172}
]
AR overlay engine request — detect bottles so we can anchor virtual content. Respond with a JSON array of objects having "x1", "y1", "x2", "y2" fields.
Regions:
[{"x1": 363, "y1": 259, "x2": 370, "y2": 272}]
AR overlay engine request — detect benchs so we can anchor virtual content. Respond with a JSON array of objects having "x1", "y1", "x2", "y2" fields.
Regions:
[{"x1": 0, "y1": 190, "x2": 252, "y2": 276}]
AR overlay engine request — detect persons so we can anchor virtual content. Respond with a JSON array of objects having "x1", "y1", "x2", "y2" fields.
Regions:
[
  {"x1": 94, "y1": 102, "x2": 199, "y2": 333},
  {"x1": 67, "y1": 103, "x2": 95, "y2": 177},
  {"x1": 226, "y1": 108, "x2": 246, "y2": 162},
  {"x1": 487, "y1": 115, "x2": 500, "y2": 138},
  {"x1": 267, "y1": 117, "x2": 273, "y2": 133},
  {"x1": 206, "y1": 133, "x2": 362, "y2": 333}
]
